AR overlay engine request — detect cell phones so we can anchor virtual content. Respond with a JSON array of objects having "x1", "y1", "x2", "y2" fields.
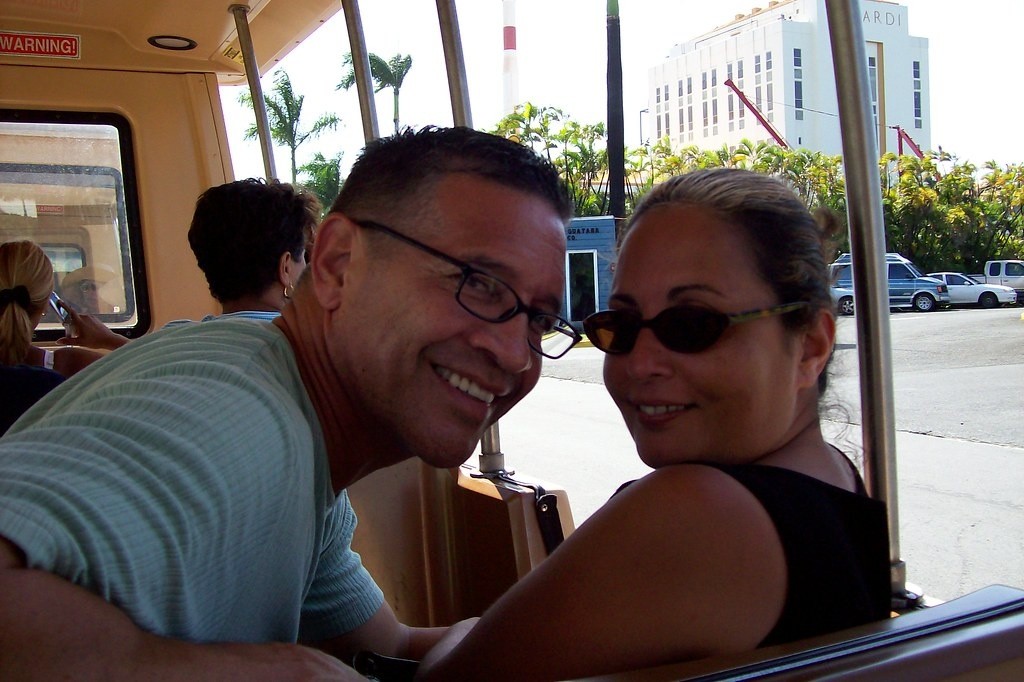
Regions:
[{"x1": 50, "y1": 291, "x2": 77, "y2": 336}]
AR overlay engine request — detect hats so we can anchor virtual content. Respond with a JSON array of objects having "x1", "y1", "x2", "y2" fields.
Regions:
[{"x1": 61, "y1": 264, "x2": 118, "y2": 308}]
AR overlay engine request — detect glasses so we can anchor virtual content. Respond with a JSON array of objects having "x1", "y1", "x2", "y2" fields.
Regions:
[
  {"x1": 582, "y1": 295, "x2": 823, "y2": 357},
  {"x1": 78, "y1": 283, "x2": 103, "y2": 294},
  {"x1": 348, "y1": 216, "x2": 581, "y2": 362}
]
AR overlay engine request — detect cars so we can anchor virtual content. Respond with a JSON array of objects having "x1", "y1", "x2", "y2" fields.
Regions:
[{"x1": 926, "y1": 270, "x2": 1017, "y2": 309}]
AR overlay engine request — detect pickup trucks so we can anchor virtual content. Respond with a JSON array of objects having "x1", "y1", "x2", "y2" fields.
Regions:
[{"x1": 964, "y1": 256, "x2": 1024, "y2": 306}]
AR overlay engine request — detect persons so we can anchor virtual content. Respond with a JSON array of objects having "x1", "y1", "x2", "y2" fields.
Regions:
[
  {"x1": 3, "y1": 118, "x2": 582, "y2": 682},
  {"x1": 159, "y1": 174, "x2": 324, "y2": 330},
  {"x1": 59, "y1": 263, "x2": 125, "y2": 313},
  {"x1": 1, "y1": 239, "x2": 136, "y2": 435},
  {"x1": 403, "y1": 168, "x2": 926, "y2": 682}
]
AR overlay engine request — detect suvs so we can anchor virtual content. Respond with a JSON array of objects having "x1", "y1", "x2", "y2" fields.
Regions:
[{"x1": 827, "y1": 250, "x2": 951, "y2": 315}]
